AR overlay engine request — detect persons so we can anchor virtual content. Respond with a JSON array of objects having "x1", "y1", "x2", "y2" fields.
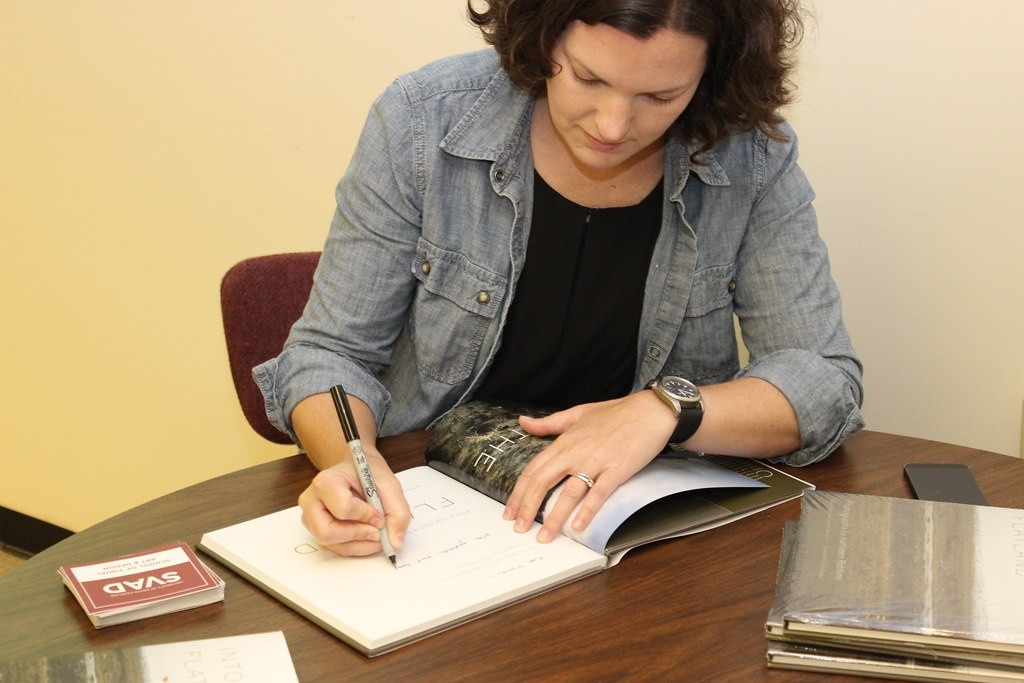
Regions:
[{"x1": 252, "y1": 0, "x2": 868, "y2": 557}]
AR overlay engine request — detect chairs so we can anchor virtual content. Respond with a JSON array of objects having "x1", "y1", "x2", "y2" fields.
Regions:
[{"x1": 220, "y1": 251, "x2": 317, "y2": 446}]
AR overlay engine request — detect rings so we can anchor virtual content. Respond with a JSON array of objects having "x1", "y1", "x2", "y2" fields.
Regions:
[{"x1": 571, "y1": 472, "x2": 595, "y2": 489}]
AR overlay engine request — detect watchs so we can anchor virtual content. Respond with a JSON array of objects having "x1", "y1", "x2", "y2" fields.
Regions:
[{"x1": 644, "y1": 373, "x2": 705, "y2": 446}]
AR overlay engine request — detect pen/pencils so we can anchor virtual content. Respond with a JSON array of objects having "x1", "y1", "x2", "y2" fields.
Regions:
[{"x1": 328, "y1": 384, "x2": 398, "y2": 568}]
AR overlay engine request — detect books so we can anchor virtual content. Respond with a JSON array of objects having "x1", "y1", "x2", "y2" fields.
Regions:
[
  {"x1": 764, "y1": 488, "x2": 1024, "y2": 683},
  {"x1": 195, "y1": 400, "x2": 816, "y2": 658}
]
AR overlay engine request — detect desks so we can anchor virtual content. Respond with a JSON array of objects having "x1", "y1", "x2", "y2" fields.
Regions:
[{"x1": 0, "y1": 431, "x2": 1024, "y2": 683}]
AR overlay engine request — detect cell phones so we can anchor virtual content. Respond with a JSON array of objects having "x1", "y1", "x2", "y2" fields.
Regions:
[{"x1": 903, "y1": 461, "x2": 989, "y2": 507}]
